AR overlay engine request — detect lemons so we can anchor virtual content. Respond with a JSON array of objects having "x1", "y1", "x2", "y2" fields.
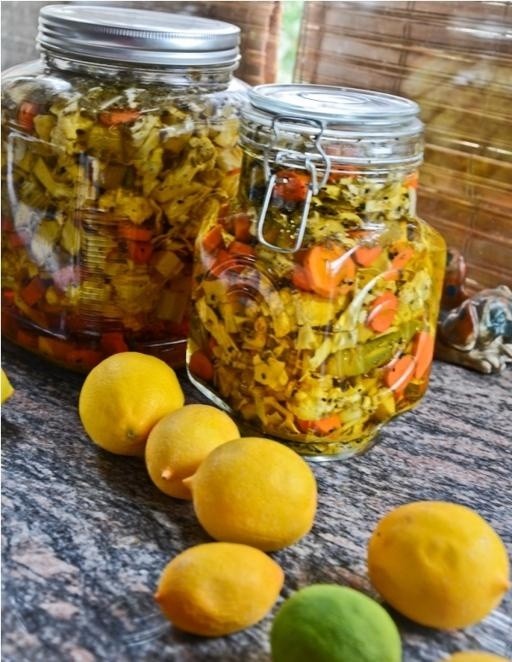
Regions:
[
  {"x1": 182, "y1": 438, "x2": 319, "y2": 553},
  {"x1": 80, "y1": 350, "x2": 187, "y2": 456},
  {"x1": 443, "y1": 650, "x2": 508, "y2": 662},
  {"x1": 368, "y1": 502, "x2": 512, "y2": 632},
  {"x1": 146, "y1": 405, "x2": 243, "y2": 501},
  {"x1": 270, "y1": 583, "x2": 403, "y2": 662},
  {"x1": 152, "y1": 541, "x2": 283, "y2": 635}
]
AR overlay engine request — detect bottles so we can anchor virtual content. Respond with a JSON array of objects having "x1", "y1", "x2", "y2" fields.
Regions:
[
  {"x1": 185, "y1": 78, "x2": 450, "y2": 467},
  {"x1": 1, "y1": 2, "x2": 250, "y2": 383}
]
